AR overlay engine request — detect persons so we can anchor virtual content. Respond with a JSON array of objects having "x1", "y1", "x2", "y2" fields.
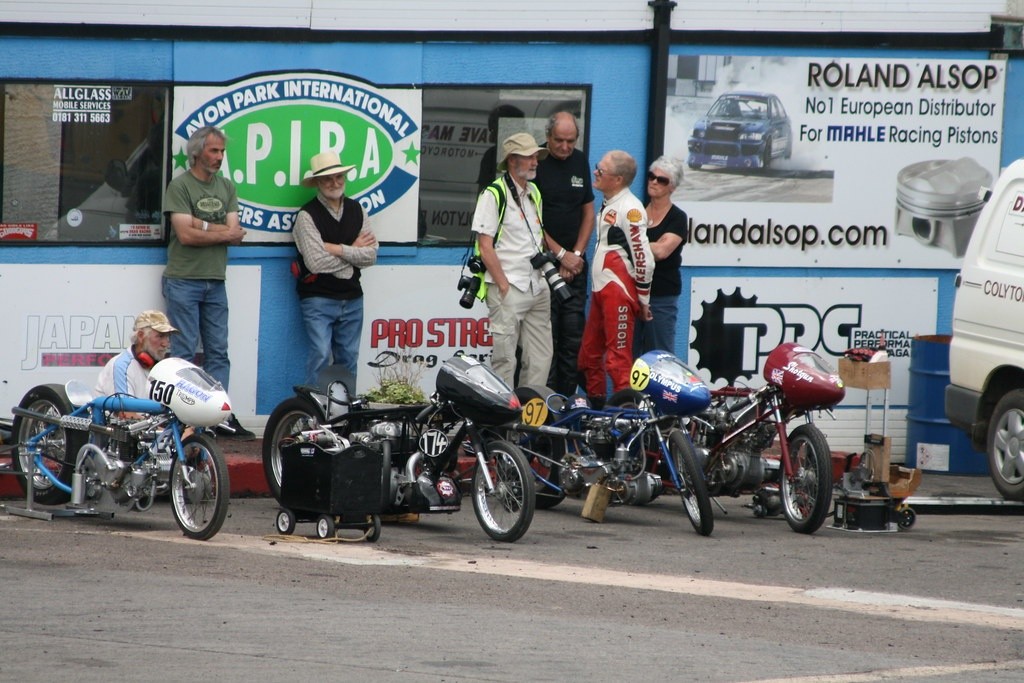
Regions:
[
  {"x1": 186, "y1": 443, "x2": 211, "y2": 495},
  {"x1": 471, "y1": 133, "x2": 553, "y2": 389},
  {"x1": 633, "y1": 157, "x2": 687, "y2": 365},
  {"x1": 291, "y1": 150, "x2": 379, "y2": 395},
  {"x1": 162, "y1": 126, "x2": 256, "y2": 442},
  {"x1": 94, "y1": 310, "x2": 208, "y2": 471},
  {"x1": 123, "y1": 126, "x2": 160, "y2": 224},
  {"x1": 576, "y1": 150, "x2": 656, "y2": 399},
  {"x1": 477, "y1": 105, "x2": 525, "y2": 194},
  {"x1": 529, "y1": 111, "x2": 595, "y2": 396}
]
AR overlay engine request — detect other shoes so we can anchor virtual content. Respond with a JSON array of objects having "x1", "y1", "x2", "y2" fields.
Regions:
[{"x1": 215, "y1": 414, "x2": 256, "y2": 441}]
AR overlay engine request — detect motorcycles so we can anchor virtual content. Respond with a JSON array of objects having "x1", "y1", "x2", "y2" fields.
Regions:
[
  {"x1": 495, "y1": 349, "x2": 715, "y2": 536},
  {"x1": 605, "y1": 343, "x2": 847, "y2": 534},
  {"x1": 259, "y1": 355, "x2": 536, "y2": 543},
  {"x1": 1, "y1": 357, "x2": 236, "y2": 541}
]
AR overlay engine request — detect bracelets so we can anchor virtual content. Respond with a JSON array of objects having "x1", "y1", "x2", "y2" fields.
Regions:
[
  {"x1": 555, "y1": 247, "x2": 566, "y2": 261},
  {"x1": 202, "y1": 220, "x2": 208, "y2": 231}
]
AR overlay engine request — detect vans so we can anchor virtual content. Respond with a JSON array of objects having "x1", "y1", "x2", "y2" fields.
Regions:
[{"x1": 944, "y1": 158, "x2": 1024, "y2": 502}]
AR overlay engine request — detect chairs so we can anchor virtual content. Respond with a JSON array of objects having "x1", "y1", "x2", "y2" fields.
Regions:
[{"x1": 725, "y1": 103, "x2": 744, "y2": 117}]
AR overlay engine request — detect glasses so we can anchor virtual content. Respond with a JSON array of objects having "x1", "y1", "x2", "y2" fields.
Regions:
[
  {"x1": 595, "y1": 163, "x2": 622, "y2": 177},
  {"x1": 647, "y1": 171, "x2": 673, "y2": 186}
]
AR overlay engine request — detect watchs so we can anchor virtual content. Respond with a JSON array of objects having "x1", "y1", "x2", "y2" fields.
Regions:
[{"x1": 573, "y1": 250, "x2": 582, "y2": 257}]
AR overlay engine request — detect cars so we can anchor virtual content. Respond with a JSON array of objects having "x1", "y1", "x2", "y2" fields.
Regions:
[{"x1": 686, "y1": 89, "x2": 793, "y2": 175}]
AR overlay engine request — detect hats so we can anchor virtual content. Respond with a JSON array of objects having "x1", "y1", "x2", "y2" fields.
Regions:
[
  {"x1": 496, "y1": 133, "x2": 550, "y2": 170},
  {"x1": 300, "y1": 151, "x2": 357, "y2": 187},
  {"x1": 133, "y1": 310, "x2": 181, "y2": 335}
]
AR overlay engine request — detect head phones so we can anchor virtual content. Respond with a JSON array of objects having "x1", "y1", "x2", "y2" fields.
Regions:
[{"x1": 131, "y1": 344, "x2": 159, "y2": 369}]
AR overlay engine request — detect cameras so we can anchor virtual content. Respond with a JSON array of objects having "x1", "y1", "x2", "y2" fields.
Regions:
[
  {"x1": 457, "y1": 275, "x2": 482, "y2": 309},
  {"x1": 530, "y1": 250, "x2": 576, "y2": 304},
  {"x1": 467, "y1": 256, "x2": 487, "y2": 274}
]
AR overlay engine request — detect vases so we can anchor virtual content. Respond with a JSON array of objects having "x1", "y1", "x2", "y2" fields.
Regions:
[{"x1": 368, "y1": 402, "x2": 415, "y2": 409}]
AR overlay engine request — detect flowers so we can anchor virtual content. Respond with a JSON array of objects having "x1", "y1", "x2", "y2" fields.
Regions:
[{"x1": 363, "y1": 345, "x2": 431, "y2": 405}]
